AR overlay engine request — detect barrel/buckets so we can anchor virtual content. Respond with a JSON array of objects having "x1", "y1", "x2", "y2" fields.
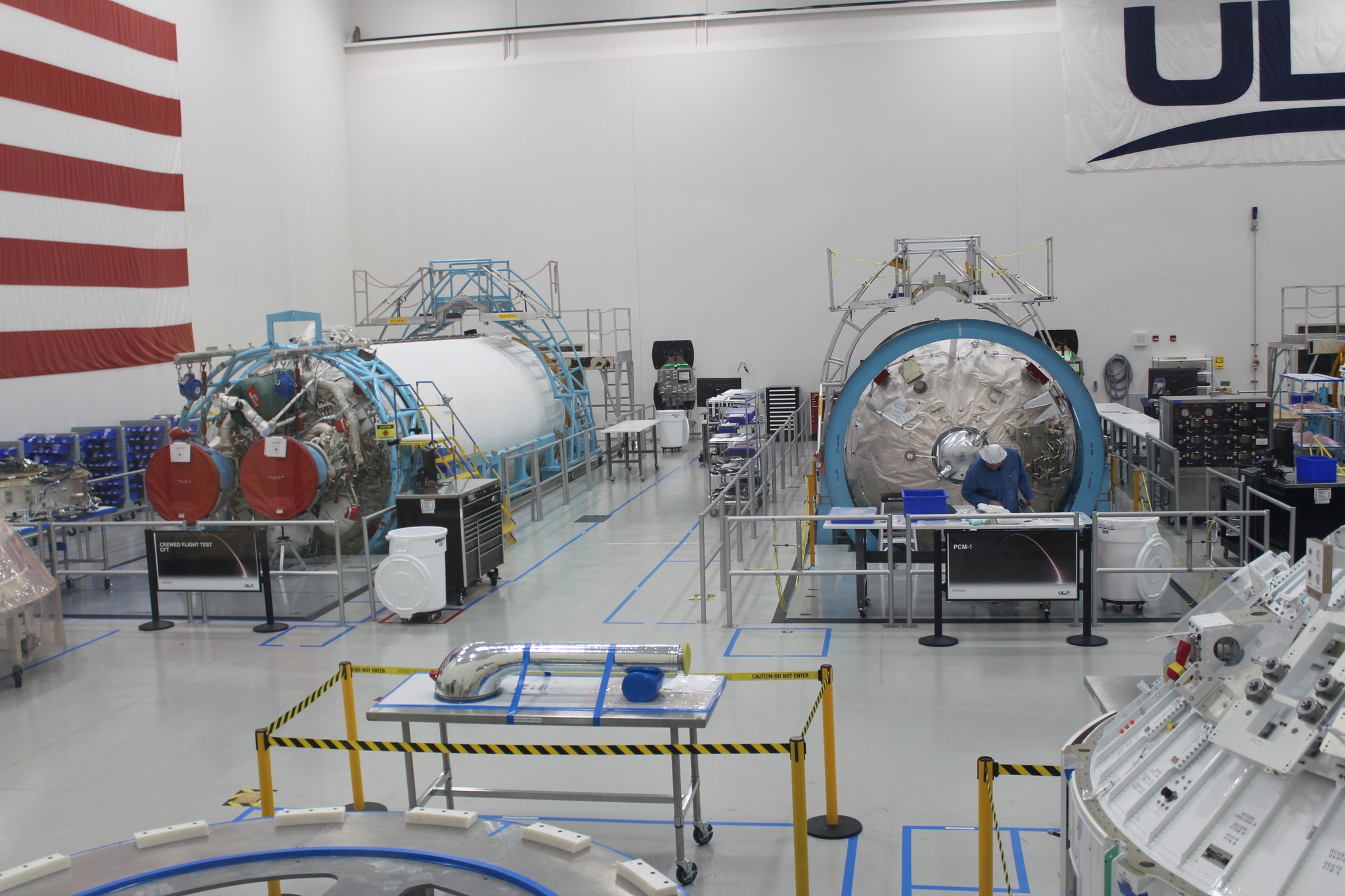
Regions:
[
  {"x1": 1097, "y1": 517, "x2": 1159, "y2": 601},
  {"x1": 657, "y1": 410, "x2": 685, "y2": 448},
  {"x1": 385, "y1": 526, "x2": 448, "y2": 615}
]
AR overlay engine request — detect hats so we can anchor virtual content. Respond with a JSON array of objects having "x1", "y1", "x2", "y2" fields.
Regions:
[{"x1": 979, "y1": 444, "x2": 1007, "y2": 464}]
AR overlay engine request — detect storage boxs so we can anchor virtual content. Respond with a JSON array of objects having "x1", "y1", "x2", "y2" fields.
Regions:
[
  {"x1": 828, "y1": 507, "x2": 877, "y2": 524},
  {"x1": 902, "y1": 489, "x2": 949, "y2": 522},
  {"x1": 717, "y1": 407, "x2": 755, "y2": 433},
  {"x1": 1295, "y1": 455, "x2": 1338, "y2": 483}
]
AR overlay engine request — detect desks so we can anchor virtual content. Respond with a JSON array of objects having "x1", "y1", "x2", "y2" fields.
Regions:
[
  {"x1": 600, "y1": 420, "x2": 663, "y2": 481},
  {"x1": 365, "y1": 673, "x2": 728, "y2": 887},
  {"x1": 13, "y1": 505, "x2": 116, "y2": 590},
  {"x1": 823, "y1": 511, "x2": 1093, "y2": 617},
  {"x1": 1093, "y1": 403, "x2": 1161, "y2": 463}
]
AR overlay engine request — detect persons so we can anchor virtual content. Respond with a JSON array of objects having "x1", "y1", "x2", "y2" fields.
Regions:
[{"x1": 961, "y1": 442, "x2": 1034, "y2": 513}]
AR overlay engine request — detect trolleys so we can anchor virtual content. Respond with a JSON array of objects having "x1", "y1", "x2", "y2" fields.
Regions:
[
  {"x1": 8, "y1": 505, "x2": 118, "y2": 591},
  {"x1": 365, "y1": 640, "x2": 729, "y2": 886},
  {"x1": 698, "y1": 405, "x2": 769, "y2": 517}
]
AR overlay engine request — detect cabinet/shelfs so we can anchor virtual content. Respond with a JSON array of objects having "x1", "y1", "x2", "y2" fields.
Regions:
[
  {"x1": 1151, "y1": 355, "x2": 1214, "y2": 395},
  {"x1": 1279, "y1": 372, "x2": 1345, "y2": 456}
]
